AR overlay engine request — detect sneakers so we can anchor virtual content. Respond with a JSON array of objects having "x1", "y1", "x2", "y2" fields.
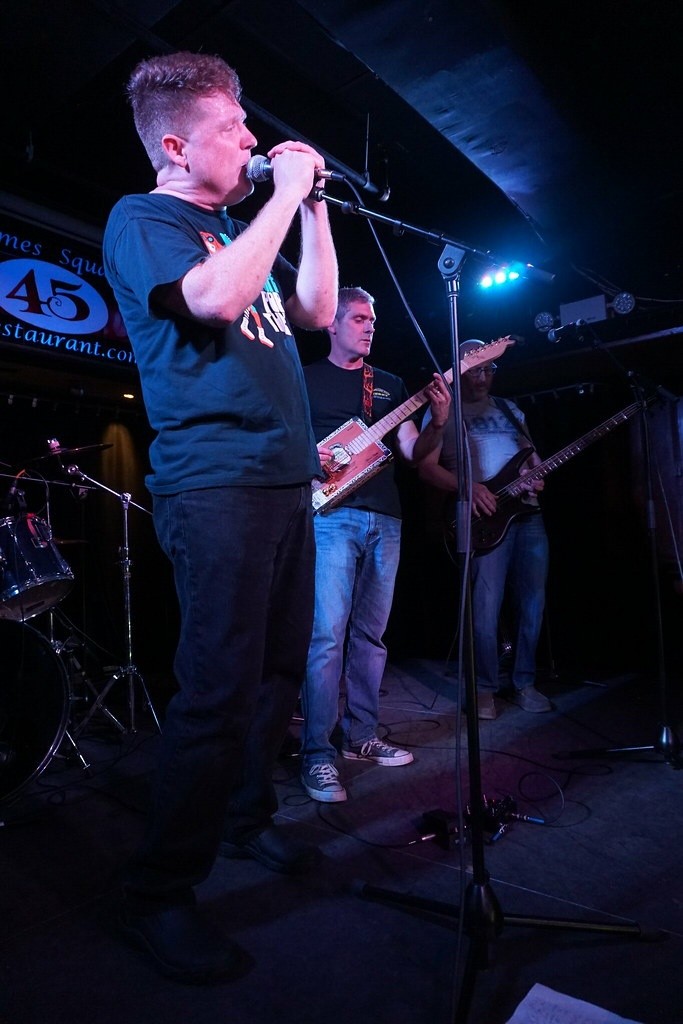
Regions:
[
  {"x1": 298, "y1": 756, "x2": 347, "y2": 803},
  {"x1": 341, "y1": 734, "x2": 414, "y2": 767}
]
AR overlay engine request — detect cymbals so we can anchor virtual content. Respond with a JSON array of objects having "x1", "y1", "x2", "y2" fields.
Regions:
[{"x1": 0, "y1": 442, "x2": 114, "y2": 472}]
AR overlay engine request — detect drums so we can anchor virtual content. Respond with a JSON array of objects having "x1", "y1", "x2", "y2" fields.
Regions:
[
  {"x1": 0, "y1": 511, "x2": 76, "y2": 623},
  {"x1": 0, "y1": 618, "x2": 72, "y2": 809}
]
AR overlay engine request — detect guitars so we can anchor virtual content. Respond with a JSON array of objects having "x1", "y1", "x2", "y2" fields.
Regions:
[
  {"x1": 441, "y1": 387, "x2": 667, "y2": 553},
  {"x1": 311, "y1": 333, "x2": 517, "y2": 516}
]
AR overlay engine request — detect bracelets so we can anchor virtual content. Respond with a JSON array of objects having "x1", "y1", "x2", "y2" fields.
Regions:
[{"x1": 431, "y1": 416, "x2": 449, "y2": 429}]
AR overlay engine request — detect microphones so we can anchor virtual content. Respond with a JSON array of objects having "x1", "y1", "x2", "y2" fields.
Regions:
[
  {"x1": 245, "y1": 154, "x2": 345, "y2": 181},
  {"x1": 4, "y1": 480, "x2": 18, "y2": 511},
  {"x1": 548, "y1": 320, "x2": 584, "y2": 342}
]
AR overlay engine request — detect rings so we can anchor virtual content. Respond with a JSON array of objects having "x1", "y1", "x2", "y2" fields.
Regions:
[{"x1": 435, "y1": 390, "x2": 440, "y2": 394}]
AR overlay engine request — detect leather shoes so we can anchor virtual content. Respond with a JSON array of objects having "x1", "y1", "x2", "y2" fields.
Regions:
[
  {"x1": 121, "y1": 903, "x2": 253, "y2": 983},
  {"x1": 504, "y1": 683, "x2": 552, "y2": 713},
  {"x1": 476, "y1": 692, "x2": 496, "y2": 719},
  {"x1": 219, "y1": 826, "x2": 312, "y2": 877}
]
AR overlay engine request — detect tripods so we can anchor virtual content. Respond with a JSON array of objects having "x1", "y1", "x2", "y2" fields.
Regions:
[
  {"x1": 56, "y1": 458, "x2": 165, "y2": 751},
  {"x1": 300, "y1": 182, "x2": 683, "y2": 1024}
]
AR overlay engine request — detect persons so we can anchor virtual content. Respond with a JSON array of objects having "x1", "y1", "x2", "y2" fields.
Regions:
[
  {"x1": 299, "y1": 287, "x2": 451, "y2": 802},
  {"x1": 415, "y1": 340, "x2": 552, "y2": 715},
  {"x1": 98, "y1": 51, "x2": 443, "y2": 983}
]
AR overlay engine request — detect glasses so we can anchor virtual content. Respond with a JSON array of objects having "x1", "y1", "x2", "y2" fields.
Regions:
[{"x1": 465, "y1": 362, "x2": 497, "y2": 377}]
